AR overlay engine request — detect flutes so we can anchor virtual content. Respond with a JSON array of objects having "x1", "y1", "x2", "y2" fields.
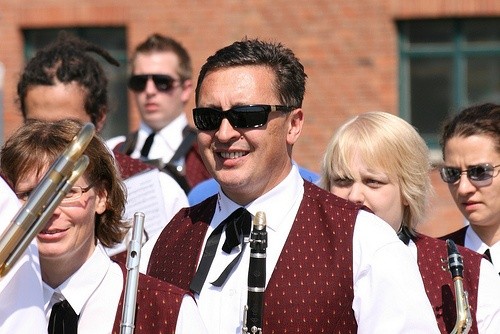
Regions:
[
  {"x1": 242, "y1": 212, "x2": 267, "y2": 333},
  {"x1": 119, "y1": 210, "x2": 146, "y2": 334}
]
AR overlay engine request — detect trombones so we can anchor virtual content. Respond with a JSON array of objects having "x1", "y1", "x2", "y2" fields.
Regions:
[{"x1": 0, "y1": 120, "x2": 96, "y2": 281}]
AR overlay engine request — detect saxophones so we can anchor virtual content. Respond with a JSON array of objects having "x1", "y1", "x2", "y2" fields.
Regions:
[{"x1": 445, "y1": 235, "x2": 472, "y2": 334}]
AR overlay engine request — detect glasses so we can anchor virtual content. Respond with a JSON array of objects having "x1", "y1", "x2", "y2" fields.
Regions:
[
  {"x1": 439, "y1": 162, "x2": 500, "y2": 184},
  {"x1": 12, "y1": 183, "x2": 94, "y2": 202},
  {"x1": 128, "y1": 73, "x2": 184, "y2": 92},
  {"x1": 192, "y1": 104, "x2": 294, "y2": 132}
]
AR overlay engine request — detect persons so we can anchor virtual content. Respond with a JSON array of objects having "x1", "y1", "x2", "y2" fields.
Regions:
[
  {"x1": 187, "y1": 163, "x2": 322, "y2": 206},
  {"x1": 103, "y1": 32, "x2": 212, "y2": 196},
  {"x1": 16, "y1": 40, "x2": 190, "y2": 274},
  {"x1": 435, "y1": 102, "x2": 500, "y2": 268},
  {"x1": 0, "y1": 117, "x2": 209, "y2": 334},
  {"x1": 146, "y1": 39, "x2": 439, "y2": 334},
  {"x1": 321, "y1": 112, "x2": 500, "y2": 334}
]
